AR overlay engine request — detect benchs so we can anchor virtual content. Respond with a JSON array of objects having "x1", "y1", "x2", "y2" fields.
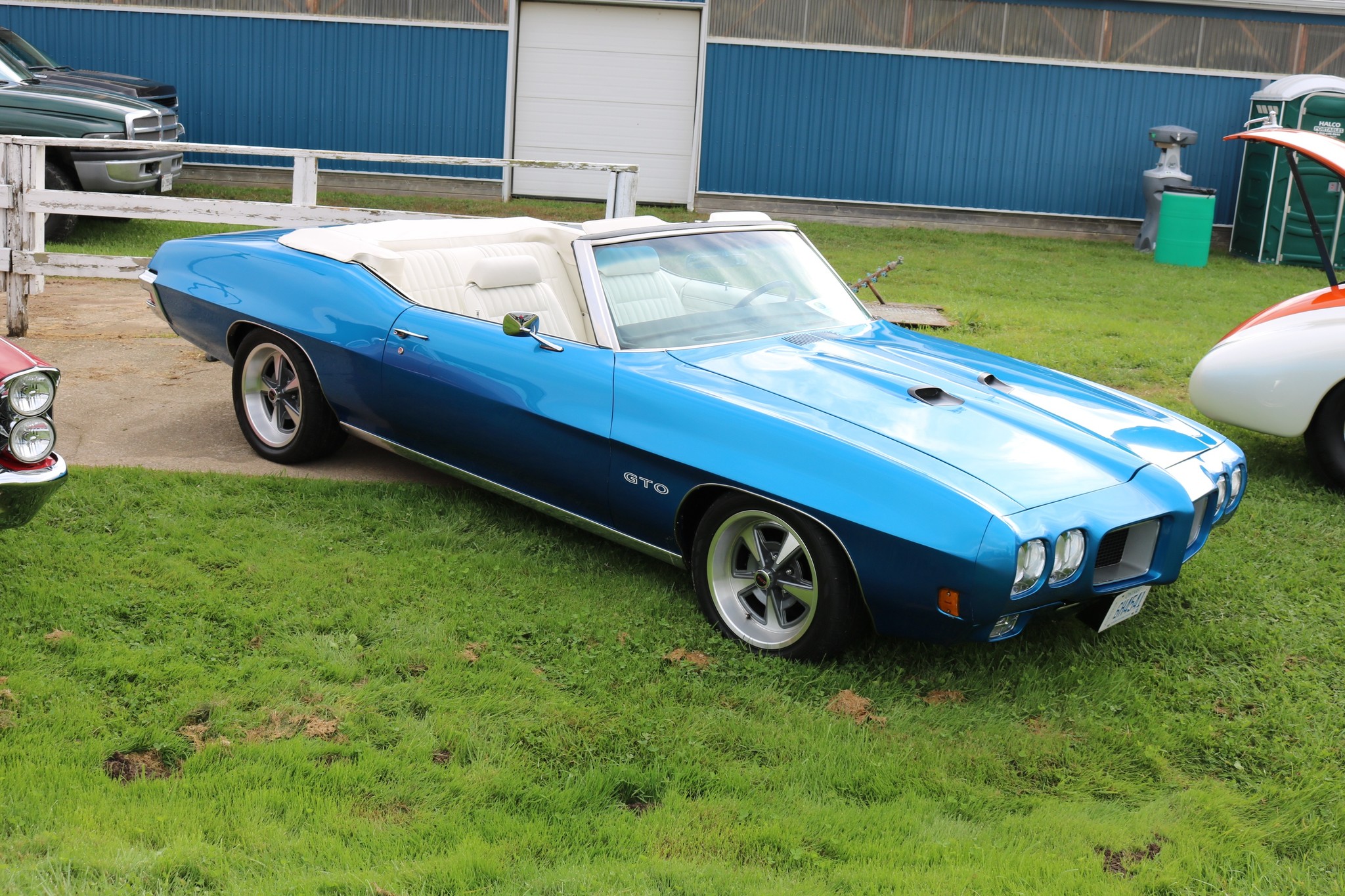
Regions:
[{"x1": 396, "y1": 242, "x2": 588, "y2": 343}]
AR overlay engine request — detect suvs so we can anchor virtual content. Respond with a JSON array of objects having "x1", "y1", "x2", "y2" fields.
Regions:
[{"x1": 0, "y1": 27, "x2": 186, "y2": 242}]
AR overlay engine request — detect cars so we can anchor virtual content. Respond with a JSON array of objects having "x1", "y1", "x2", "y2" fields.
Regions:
[
  {"x1": 1187, "y1": 124, "x2": 1345, "y2": 493},
  {"x1": 1, "y1": 338, "x2": 69, "y2": 531},
  {"x1": 138, "y1": 212, "x2": 1254, "y2": 662}
]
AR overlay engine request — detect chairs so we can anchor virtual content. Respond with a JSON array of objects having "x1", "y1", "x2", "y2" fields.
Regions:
[
  {"x1": 596, "y1": 245, "x2": 683, "y2": 327},
  {"x1": 464, "y1": 255, "x2": 578, "y2": 340}
]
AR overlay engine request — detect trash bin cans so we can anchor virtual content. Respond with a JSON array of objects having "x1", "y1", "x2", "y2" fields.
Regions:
[
  {"x1": 1153, "y1": 185, "x2": 1217, "y2": 268},
  {"x1": 1132, "y1": 124, "x2": 1199, "y2": 253}
]
